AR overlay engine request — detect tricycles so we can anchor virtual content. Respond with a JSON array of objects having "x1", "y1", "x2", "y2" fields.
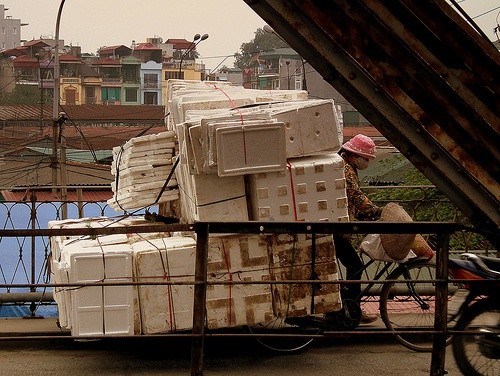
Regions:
[{"x1": 246, "y1": 200, "x2": 463, "y2": 354}]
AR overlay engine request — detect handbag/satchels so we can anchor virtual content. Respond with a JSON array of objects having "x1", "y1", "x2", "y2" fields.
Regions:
[{"x1": 359, "y1": 232, "x2": 417, "y2": 263}]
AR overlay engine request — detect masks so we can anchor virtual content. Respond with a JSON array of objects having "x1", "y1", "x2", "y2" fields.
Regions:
[{"x1": 353, "y1": 156, "x2": 369, "y2": 170}]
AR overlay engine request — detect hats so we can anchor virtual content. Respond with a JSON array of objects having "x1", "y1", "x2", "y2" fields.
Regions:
[
  {"x1": 341, "y1": 134, "x2": 377, "y2": 160},
  {"x1": 381, "y1": 203, "x2": 416, "y2": 260}
]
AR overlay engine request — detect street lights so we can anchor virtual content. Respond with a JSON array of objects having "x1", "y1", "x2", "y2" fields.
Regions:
[{"x1": 176, "y1": 32, "x2": 209, "y2": 79}]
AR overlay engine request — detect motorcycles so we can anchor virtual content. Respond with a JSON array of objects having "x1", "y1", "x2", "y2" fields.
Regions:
[{"x1": 445, "y1": 248, "x2": 500, "y2": 373}]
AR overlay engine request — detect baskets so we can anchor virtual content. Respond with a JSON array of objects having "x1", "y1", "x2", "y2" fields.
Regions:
[{"x1": 411, "y1": 234, "x2": 434, "y2": 262}]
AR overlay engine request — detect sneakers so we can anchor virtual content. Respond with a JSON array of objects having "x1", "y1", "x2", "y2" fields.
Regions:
[{"x1": 349, "y1": 309, "x2": 377, "y2": 322}]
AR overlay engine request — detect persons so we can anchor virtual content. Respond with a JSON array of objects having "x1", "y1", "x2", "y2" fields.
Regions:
[{"x1": 334, "y1": 134, "x2": 385, "y2": 324}]
